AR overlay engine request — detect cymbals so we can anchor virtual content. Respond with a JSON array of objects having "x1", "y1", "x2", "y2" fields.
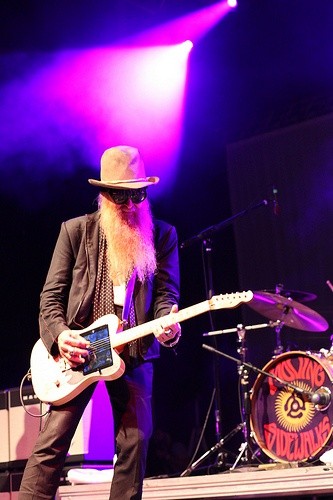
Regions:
[{"x1": 245, "y1": 285, "x2": 329, "y2": 332}]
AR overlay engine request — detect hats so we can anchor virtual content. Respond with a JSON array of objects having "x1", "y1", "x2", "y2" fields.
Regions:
[{"x1": 88, "y1": 146, "x2": 160, "y2": 190}]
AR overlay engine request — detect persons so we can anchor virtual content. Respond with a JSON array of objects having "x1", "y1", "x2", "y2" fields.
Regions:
[{"x1": 17, "y1": 145, "x2": 181, "y2": 500}]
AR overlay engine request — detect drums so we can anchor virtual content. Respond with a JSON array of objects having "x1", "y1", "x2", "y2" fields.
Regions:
[{"x1": 248, "y1": 348, "x2": 333, "y2": 466}]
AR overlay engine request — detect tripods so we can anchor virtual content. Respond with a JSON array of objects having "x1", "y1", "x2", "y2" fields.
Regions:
[{"x1": 151, "y1": 199, "x2": 281, "y2": 479}]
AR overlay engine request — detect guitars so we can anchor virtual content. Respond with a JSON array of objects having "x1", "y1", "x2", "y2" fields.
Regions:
[{"x1": 30, "y1": 289, "x2": 255, "y2": 406}]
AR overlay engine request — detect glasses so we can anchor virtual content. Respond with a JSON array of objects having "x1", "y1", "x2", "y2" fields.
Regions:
[{"x1": 107, "y1": 189, "x2": 147, "y2": 205}]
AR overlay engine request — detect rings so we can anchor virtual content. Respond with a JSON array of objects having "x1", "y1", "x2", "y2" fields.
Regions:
[
  {"x1": 69, "y1": 347, "x2": 74, "y2": 355},
  {"x1": 164, "y1": 329, "x2": 173, "y2": 334}
]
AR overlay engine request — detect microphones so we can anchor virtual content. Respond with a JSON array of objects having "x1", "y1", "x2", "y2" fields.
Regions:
[
  {"x1": 295, "y1": 388, "x2": 326, "y2": 405},
  {"x1": 273, "y1": 185, "x2": 280, "y2": 215}
]
